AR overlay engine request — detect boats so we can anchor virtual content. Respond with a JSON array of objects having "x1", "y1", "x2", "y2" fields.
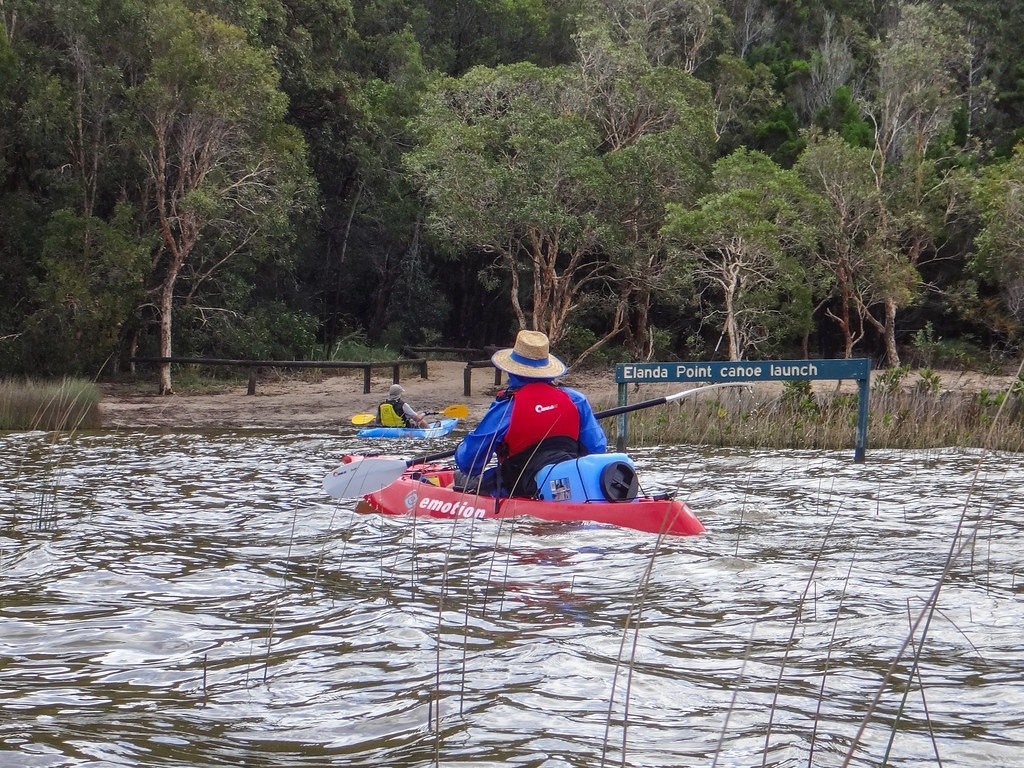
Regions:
[
  {"x1": 357, "y1": 416, "x2": 459, "y2": 438},
  {"x1": 343, "y1": 451, "x2": 707, "y2": 537}
]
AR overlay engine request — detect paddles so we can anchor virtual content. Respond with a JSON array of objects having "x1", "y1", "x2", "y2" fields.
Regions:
[
  {"x1": 323, "y1": 382, "x2": 749, "y2": 498},
  {"x1": 351, "y1": 404, "x2": 469, "y2": 425}
]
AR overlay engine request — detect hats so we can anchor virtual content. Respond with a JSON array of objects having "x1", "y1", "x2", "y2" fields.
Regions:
[
  {"x1": 491, "y1": 329, "x2": 567, "y2": 379},
  {"x1": 389, "y1": 384, "x2": 405, "y2": 396}
]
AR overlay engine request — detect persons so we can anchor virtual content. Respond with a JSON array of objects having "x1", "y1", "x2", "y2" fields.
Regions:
[
  {"x1": 451, "y1": 330, "x2": 605, "y2": 498},
  {"x1": 378, "y1": 381, "x2": 433, "y2": 430}
]
